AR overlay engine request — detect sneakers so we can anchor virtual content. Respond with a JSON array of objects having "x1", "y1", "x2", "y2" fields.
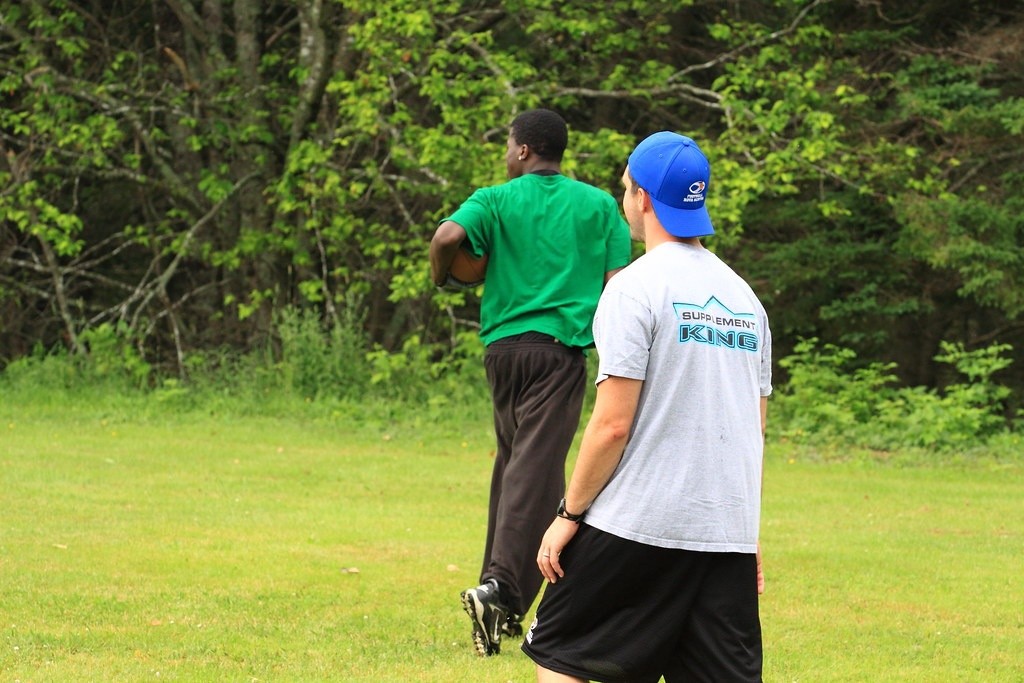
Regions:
[
  {"x1": 501, "y1": 612, "x2": 522, "y2": 638},
  {"x1": 460, "y1": 578, "x2": 510, "y2": 658}
]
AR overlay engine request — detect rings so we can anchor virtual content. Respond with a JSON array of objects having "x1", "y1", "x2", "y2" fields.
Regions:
[{"x1": 542, "y1": 551, "x2": 548, "y2": 558}]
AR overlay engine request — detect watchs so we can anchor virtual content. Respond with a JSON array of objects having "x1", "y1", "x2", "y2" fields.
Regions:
[{"x1": 554, "y1": 497, "x2": 591, "y2": 526}]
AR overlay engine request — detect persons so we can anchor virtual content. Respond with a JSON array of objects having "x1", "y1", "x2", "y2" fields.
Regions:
[
  {"x1": 518, "y1": 128, "x2": 779, "y2": 682},
  {"x1": 429, "y1": 107, "x2": 631, "y2": 658}
]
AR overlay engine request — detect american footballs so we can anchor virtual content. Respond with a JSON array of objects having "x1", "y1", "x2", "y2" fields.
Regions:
[{"x1": 448, "y1": 247, "x2": 487, "y2": 286}]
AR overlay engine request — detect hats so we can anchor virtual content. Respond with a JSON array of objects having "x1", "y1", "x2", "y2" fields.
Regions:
[{"x1": 628, "y1": 130, "x2": 716, "y2": 238}]
